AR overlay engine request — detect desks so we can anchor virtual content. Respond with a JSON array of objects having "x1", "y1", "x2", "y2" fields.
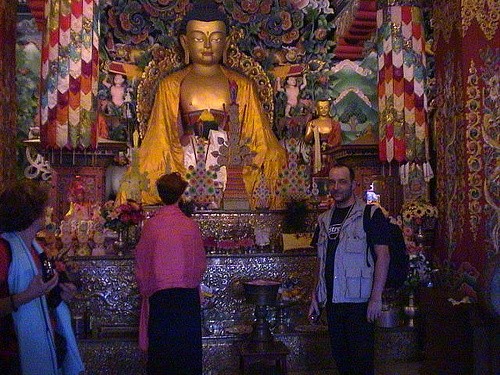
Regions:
[{"x1": 69, "y1": 253, "x2": 318, "y2": 338}]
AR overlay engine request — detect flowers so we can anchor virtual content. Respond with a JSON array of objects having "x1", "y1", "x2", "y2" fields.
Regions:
[
  {"x1": 397, "y1": 199, "x2": 439, "y2": 253},
  {"x1": 99, "y1": 199, "x2": 147, "y2": 232}
]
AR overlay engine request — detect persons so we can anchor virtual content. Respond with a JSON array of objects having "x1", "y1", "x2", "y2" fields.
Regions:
[
  {"x1": 106, "y1": 0, "x2": 292, "y2": 213},
  {"x1": 129, "y1": 171, "x2": 212, "y2": 375},
  {"x1": 305, "y1": 163, "x2": 392, "y2": 375},
  {"x1": 303, "y1": 100, "x2": 344, "y2": 201},
  {"x1": 0, "y1": 179, "x2": 88, "y2": 375}
]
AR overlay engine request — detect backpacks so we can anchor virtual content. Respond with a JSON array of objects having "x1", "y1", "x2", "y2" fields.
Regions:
[{"x1": 363, "y1": 203, "x2": 409, "y2": 290}]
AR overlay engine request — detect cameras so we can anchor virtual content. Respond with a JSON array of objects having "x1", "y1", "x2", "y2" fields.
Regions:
[
  {"x1": 43, "y1": 259, "x2": 54, "y2": 281},
  {"x1": 308, "y1": 307, "x2": 325, "y2": 323}
]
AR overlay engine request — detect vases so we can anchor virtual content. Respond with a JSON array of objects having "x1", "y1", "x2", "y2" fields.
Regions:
[
  {"x1": 416, "y1": 226, "x2": 425, "y2": 241},
  {"x1": 114, "y1": 230, "x2": 127, "y2": 257}
]
